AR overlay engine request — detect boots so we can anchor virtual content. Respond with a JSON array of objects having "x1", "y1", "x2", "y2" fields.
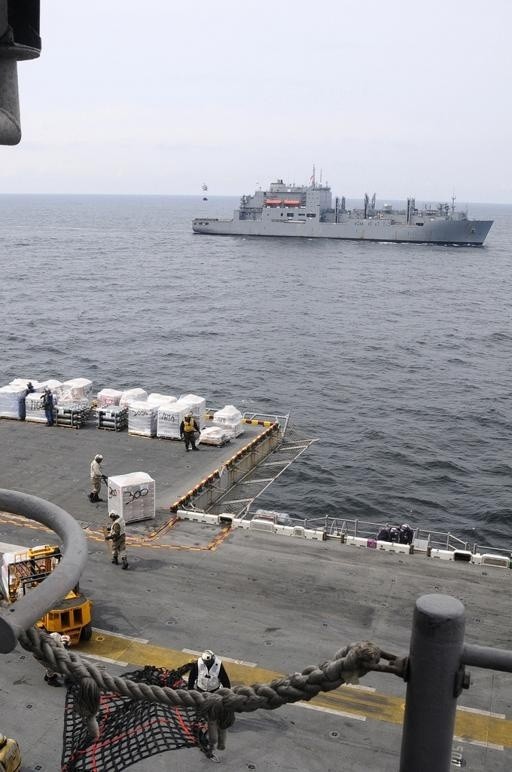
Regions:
[
  {"x1": 46, "y1": 420, "x2": 54, "y2": 426},
  {"x1": 112, "y1": 556, "x2": 118, "y2": 564},
  {"x1": 44, "y1": 673, "x2": 61, "y2": 687},
  {"x1": 122, "y1": 558, "x2": 128, "y2": 569},
  {"x1": 91, "y1": 493, "x2": 103, "y2": 502}
]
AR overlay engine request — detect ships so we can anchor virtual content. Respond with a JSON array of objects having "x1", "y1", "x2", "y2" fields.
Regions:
[{"x1": 191, "y1": 162, "x2": 499, "y2": 249}]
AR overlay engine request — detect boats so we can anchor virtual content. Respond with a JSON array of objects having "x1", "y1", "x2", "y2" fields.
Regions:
[{"x1": 201, "y1": 197, "x2": 208, "y2": 201}]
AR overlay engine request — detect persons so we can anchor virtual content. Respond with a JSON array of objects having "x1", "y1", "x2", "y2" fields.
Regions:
[
  {"x1": 40, "y1": 387, "x2": 55, "y2": 427},
  {"x1": 103, "y1": 510, "x2": 129, "y2": 569},
  {"x1": 44, "y1": 631, "x2": 71, "y2": 688},
  {"x1": 0, "y1": 732, "x2": 22, "y2": 771},
  {"x1": 188, "y1": 650, "x2": 231, "y2": 693},
  {"x1": 179, "y1": 414, "x2": 201, "y2": 452},
  {"x1": 87, "y1": 453, "x2": 108, "y2": 503}
]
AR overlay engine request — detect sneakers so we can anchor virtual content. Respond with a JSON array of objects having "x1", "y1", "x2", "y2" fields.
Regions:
[
  {"x1": 192, "y1": 447, "x2": 199, "y2": 450},
  {"x1": 186, "y1": 448, "x2": 188, "y2": 452}
]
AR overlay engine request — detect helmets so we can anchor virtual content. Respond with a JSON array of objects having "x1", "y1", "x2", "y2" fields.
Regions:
[
  {"x1": 95, "y1": 454, "x2": 104, "y2": 461},
  {"x1": 0, "y1": 733, "x2": 8, "y2": 746},
  {"x1": 44, "y1": 387, "x2": 50, "y2": 393},
  {"x1": 184, "y1": 413, "x2": 191, "y2": 417},
  {"x1": 60, "y1": 634, "x2": 71, "y2": 647},
  {"x1": 26, "y1": 382, "x2": 32, "y2": 388},
  {"x1": 202, "y1": 649, "x2": 214, "y2": 661}
]
